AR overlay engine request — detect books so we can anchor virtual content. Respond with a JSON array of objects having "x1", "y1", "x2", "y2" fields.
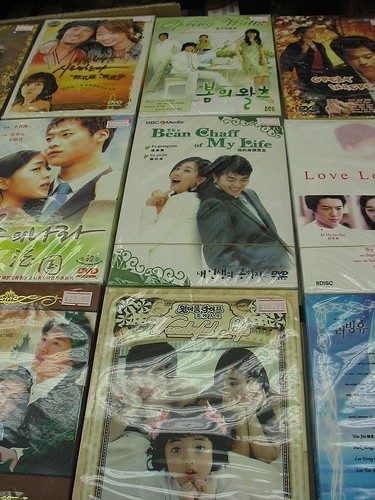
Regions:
[
  {"x1": 0, "y1": 279, "x2": 107, "y2": 500},
  {"x1": 136, "y1": 14, "x2": 281, "y2": 117},
  {"x1": 304, "y1": 290, "x2": 375, "y2": 500},
  {"x1": 0, "y1": 2, "x2": 155, "y2": 119},
  {"x1": 281, "y1": 115, "x2": 375, "y2": 295},
  {"x1": 107, "y1": 115, "x2": 299, "y2": 288},
  {"x1": 70, "y1": 287, "x2": 314, "y2": 500},
  {"x1": 271, "y1": 10, "x2": 375, "y2": 119},
  {"x1": 1, "y1": 15, "x2": 41, "y2": 117},
  {"x1": 0, "y1": 112, "x2": 134, "y2": 288}
]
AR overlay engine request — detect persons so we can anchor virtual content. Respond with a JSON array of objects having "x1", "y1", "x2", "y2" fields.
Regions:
[
  {"x1": 314, "y1": 33, "x2": 375, "y2": 117},
  {"x1": 0, "y1": 304, "x2": 94, "y2": 473},
  {"x1": 20, "y1": 115, "x2": 117, "y2": 240},
  {"x1": 235, "y1": 28, "x2": 271, "y2": 102},
  {"x1": 35, "y1": 18, "x2": 142, "y2": 69},
  {"x1": 0, "y1": 363, "x2": 35, "y2": 473},
  {"x1": 196, "y1": 154, "x2": 296, "y2": 286},
  {"x1": 99, "y1": 400, "x2": 287, "y2": 500},
  {"x1": 297, "y1": 193, "x2": 353, "y2": 235},
  {"x1": 8, "y1": 72, "x2": 58, "y2": 118},
  {"x1": 192, "y1": 34, "x2": 214, "y2": 71},
  {"x1": 0, "y1": 148, "x2": 125, "y2": 259},
  {"x1": 103, "y1": 340, "x2": 200, "y2": 440},
  {"x1": 147, "y1": 33, "x2": 177, "y2": 95},
  {"x1": 277, "y1": 26, "x2": 334, "y2": 117},
  {"x1": 202, "y1": 347, "x2": 287, "y2": 468},
  {"x1": 167, "y1": 41, "x2": 239, "y2": 102},
  {"x1": 26, "y1": 21, "x2": 100, "y2": 68},
  {"x1": 356, "y1": 194, "x2": 374, "y2": 232},
  {"x1": 125, "y1": 155, "x2": 216, "y2": 284}
]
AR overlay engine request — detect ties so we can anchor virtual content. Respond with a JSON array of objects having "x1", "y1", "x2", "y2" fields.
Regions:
[{"x1": 38, "y1": 182, "x2": 71, "y2": 225}]
